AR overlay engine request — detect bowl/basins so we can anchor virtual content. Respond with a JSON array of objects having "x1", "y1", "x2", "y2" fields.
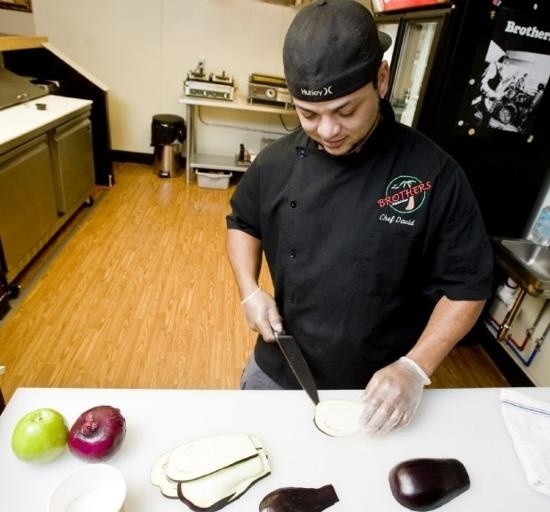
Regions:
[{"x1": 46, "y1": 463, "x2": 126, "y2": 508}]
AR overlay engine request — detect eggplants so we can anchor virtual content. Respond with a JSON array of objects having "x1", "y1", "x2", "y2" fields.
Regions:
[
  {"x1": 149, "y1": 431, "x2": 271, "y2": 512},
  {"x1": 313, "y1": 399, "x2": 365, "y2": 438},
  {"x1": 389, "y1": 458, "x2": 471, "y2": 511},
  {"x1": 259, "y1": 484, "x2": 339, "y2": 512}
]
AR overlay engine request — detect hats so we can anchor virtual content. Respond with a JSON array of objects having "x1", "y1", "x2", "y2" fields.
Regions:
[{"x1": 284, "y1": 1, "x2": 392, "y2": 102}]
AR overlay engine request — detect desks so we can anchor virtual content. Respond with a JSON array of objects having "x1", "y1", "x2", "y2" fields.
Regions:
[{"x1": 178, "y1": 93, "x2": 298, "y2": 186}]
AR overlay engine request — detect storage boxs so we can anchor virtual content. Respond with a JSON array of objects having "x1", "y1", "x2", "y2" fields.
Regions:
[{"x1": 195, "y1": 169, "x2": 233, "y2": 190}]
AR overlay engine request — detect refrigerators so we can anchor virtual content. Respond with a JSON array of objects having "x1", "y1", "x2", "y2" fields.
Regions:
[{"x1": 0, "y1": 110, "x2": 94, "y2": 286}]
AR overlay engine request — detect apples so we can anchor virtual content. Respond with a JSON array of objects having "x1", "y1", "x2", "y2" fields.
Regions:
[{"x1": 12, "y1": 408, "x2": 68, "y2": 464}]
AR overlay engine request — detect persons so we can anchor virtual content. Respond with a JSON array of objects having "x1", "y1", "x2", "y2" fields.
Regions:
[
  {"x1": 225, "y1": 0, "x2": 495, "y2": 439},
  {"x1": 468, "y1": 49, "x2": 544, "y2": 133}
]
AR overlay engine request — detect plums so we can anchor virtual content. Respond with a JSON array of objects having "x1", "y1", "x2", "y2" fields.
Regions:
[{"x1": 67, "y1": 405, "x2": 126, "y2": 463}]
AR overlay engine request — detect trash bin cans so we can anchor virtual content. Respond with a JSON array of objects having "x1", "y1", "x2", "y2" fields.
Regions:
[{"x1": 152, "y1": 114, "x2": 185, "y2": 178}]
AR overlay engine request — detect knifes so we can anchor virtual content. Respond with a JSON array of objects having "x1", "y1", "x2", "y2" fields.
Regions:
[{"x1": 274, "y1": 325, "x2": 319, "y2": 405}]
